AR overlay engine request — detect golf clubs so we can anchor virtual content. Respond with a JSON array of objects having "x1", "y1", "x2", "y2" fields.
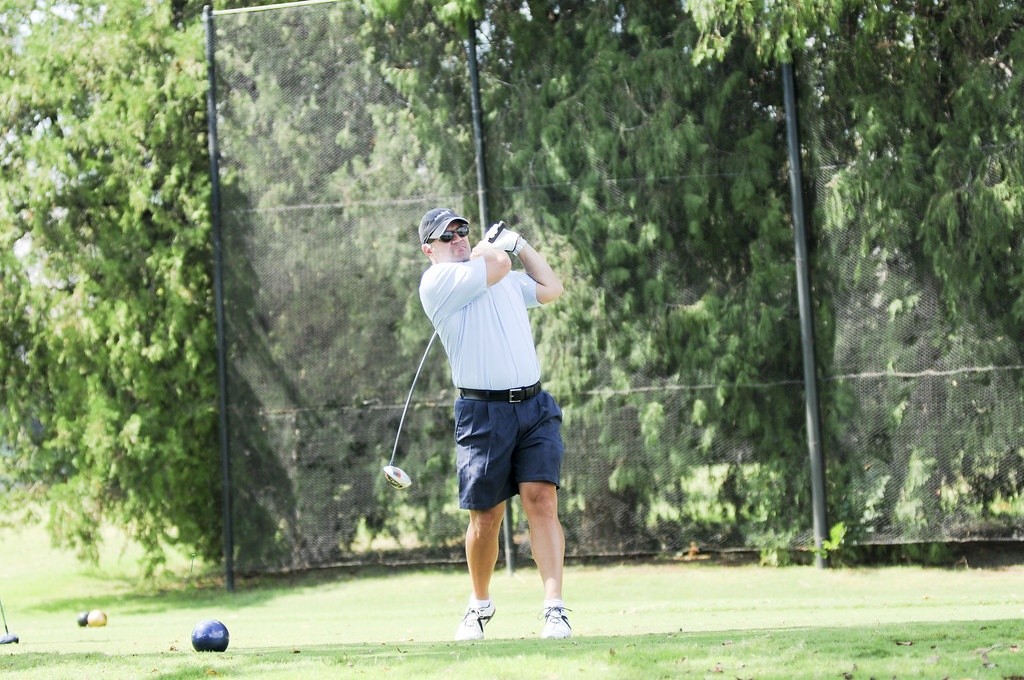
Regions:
[
  {"x1": 0, "y1": 600, "x2": 19, "y2": 644},
  {"x1": 383, "y1": 218, "x2": 506, "y2": 490}
]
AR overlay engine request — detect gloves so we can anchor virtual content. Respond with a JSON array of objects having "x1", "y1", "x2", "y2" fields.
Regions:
[{"x1": 483, "y1": 224, "x2": 528, "y2": 257}]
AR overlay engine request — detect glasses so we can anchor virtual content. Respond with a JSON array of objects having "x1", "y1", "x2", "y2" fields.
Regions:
[{"x1": 439, "y1": 225, "x2": 469, "y2": 243}]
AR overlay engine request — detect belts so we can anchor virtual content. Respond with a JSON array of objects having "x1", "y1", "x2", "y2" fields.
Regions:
[{"x1": 458, "y1": 381, "x2": 542, "y2": 403}]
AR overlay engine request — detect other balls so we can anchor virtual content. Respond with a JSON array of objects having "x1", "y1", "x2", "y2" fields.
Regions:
[
  {"x1": 191, "y1": 619, "x2": 230, "y2": 652},
  {"x1": 78, "y1": 609, "x2": 107, "y2": 628}
]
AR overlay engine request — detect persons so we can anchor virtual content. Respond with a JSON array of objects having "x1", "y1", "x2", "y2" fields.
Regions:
[{"x1": 418, "y1": 208, "x2": 573, "y2": 641}]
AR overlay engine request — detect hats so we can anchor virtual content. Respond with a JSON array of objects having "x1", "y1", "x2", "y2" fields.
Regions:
[{"x1": 418, "y1": 207, "x2": 470, "y2": 244}]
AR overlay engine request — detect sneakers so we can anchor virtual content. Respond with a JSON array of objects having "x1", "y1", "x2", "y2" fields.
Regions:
[
  {"x1": 454, "y1": 601, "x2": 497, "y2": 642},
  {"x1": 537, "y1": 605, "x2": 574, "y2": 638}
]
